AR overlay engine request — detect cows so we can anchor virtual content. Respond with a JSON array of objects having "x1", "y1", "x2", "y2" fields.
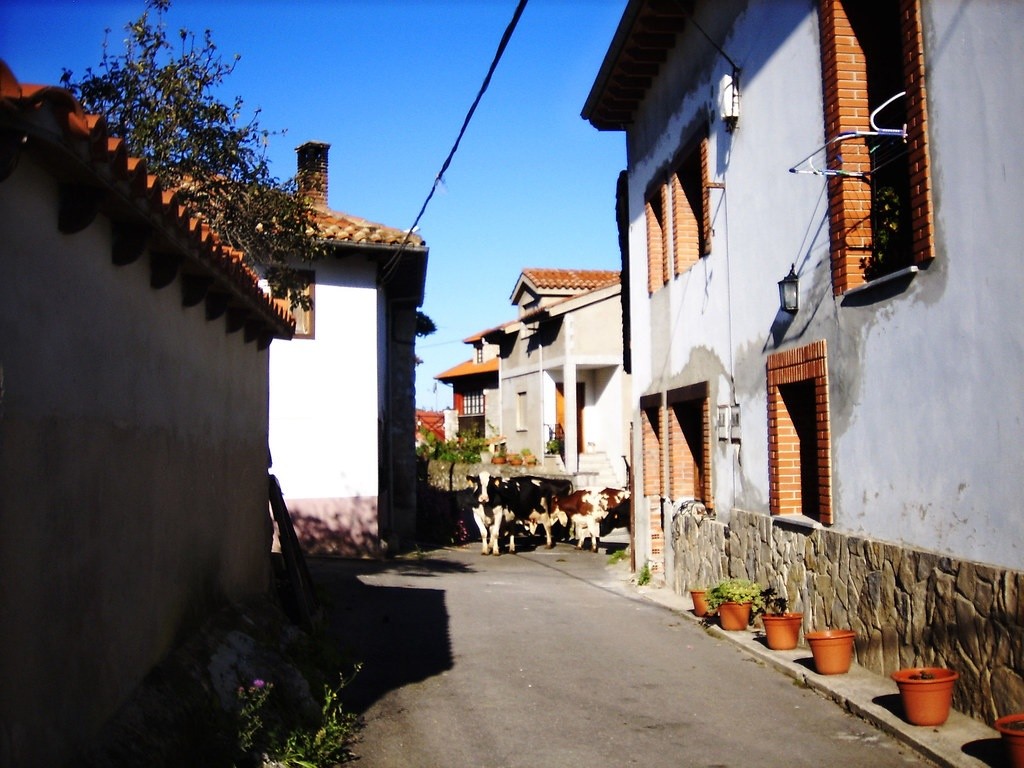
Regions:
[{"x1": 465, "y1": 471, "x2": 632, "y2": 557}]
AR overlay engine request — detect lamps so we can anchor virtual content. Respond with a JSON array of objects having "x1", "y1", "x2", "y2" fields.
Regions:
[{"x1": 778, "y1": 263, "x2": 798, "y2": 315}]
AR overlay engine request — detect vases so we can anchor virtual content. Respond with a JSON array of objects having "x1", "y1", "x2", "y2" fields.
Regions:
[
  {"x1": 995, "y1": 714, "x2": 1024, "y2": 768},
  {"x1": 805, "y1": 630, "x2": 856, "y2": 675},
  {"x1": 691, "y1": 588, "x2": 709, "y2": 617}
]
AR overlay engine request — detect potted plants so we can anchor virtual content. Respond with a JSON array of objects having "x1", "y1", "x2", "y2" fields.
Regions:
[
  {"x1": 509, "y1": 453, "x2": 524, "y2": 465},
  {"x1": 759, "y1": 588, "x2": 803, "y2": 650},
  {"x1": 703, "y1": 578, "x2": 765, "y2": 630},
  {"x1": 491, "y1": 447, "x2": 507, "y2": 464},
  {"x1": 891, "y1": 667, "x2": 959, "y2": 726}
]
